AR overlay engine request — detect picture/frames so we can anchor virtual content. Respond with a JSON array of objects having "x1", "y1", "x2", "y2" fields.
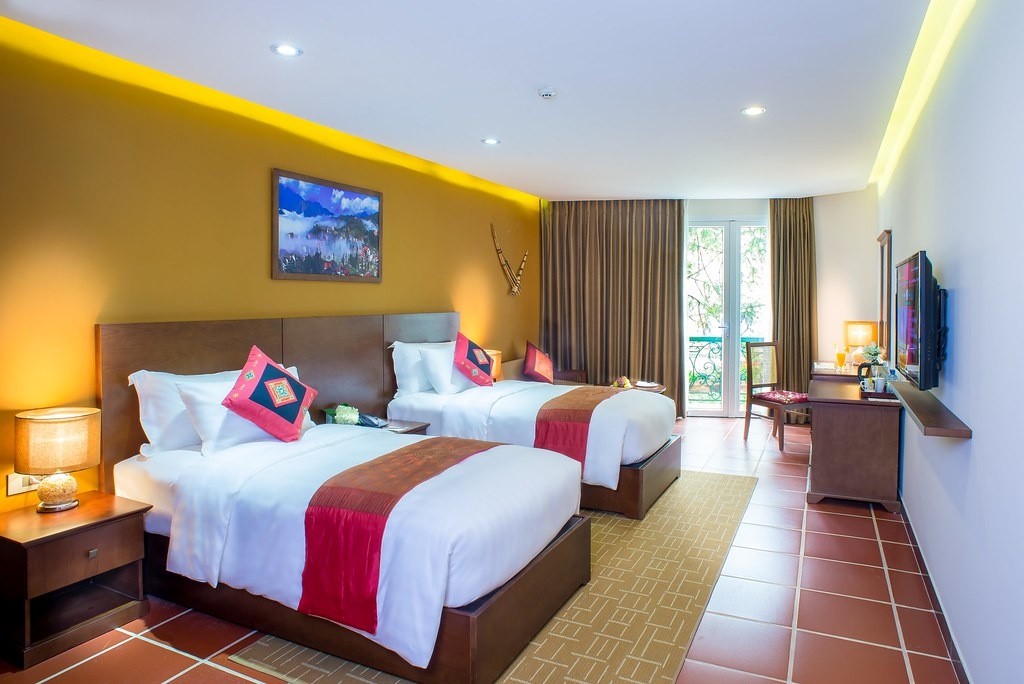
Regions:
[{"x1": 270, "y1": 168, "x2": 384, "y2": 282}]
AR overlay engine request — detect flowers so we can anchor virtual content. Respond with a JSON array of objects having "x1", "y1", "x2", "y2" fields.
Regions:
[
  {"x1": 614, "y1": 376, "x2": 630, "y2": 388},
  {"x1": 320, "y1": 402, "x2": 363, "y2": 425}
]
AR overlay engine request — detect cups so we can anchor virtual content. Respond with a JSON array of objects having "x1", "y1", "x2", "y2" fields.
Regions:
[
  {"x1": 874, "y1": 378, "x2": 886, "y2": 393},
  {"x1": 860, "y1": 377, "x2": 875, "y2": 392}
]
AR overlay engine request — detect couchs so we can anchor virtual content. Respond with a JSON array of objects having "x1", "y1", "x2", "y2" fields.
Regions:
[{"x1": 500, "y1": 353, "x2": 587, "y2": 385}]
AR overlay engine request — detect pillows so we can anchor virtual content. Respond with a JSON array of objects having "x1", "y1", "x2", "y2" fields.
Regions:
[
  {"x1": 175, "y1": 366, "x2": 317, "y2": 453},
  {"x1": 219, "y1": 344, "x2": 318, "y2": 442},
  {"x1": 522, "y1": 341, "x2": 554, "y2": 384},
  {"x1": 122, "y1": 368, "x2": 234, "y2": 455}
]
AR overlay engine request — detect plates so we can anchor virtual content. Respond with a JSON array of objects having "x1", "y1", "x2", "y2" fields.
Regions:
[{"x1": 635, "y1": 383, "x2": 658, "y2": 388}]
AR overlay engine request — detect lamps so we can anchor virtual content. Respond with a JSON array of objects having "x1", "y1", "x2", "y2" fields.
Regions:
[
  {"x1": 481, "y1": 350, "x2": 502, "y2": 381},
  {"x1": 844, "y1": 321, "x2": 878, "y2": 347},
  {"x1": 15, "y1": 406, "x2": 102, "y2": 512}
]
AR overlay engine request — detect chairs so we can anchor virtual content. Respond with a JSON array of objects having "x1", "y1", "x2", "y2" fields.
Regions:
[
  {"x1": 744, "y1": 342, "x2": 809, "y2": 451},
  {"x1": 878, "y1": 229, "x2": 891, "y2": 357}
]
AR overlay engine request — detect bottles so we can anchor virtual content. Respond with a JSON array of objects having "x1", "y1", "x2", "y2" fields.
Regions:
[{"x1": 885, "y1": 369, "x2": 898, "y2": 393}]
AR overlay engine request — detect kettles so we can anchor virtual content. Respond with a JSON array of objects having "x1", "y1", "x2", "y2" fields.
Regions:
[{"x1": 858, "y1": 362, "x2": 887, "y2": 389}]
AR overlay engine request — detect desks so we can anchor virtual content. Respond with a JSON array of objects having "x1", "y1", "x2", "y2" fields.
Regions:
[
  {"x1": 807, "y1": 379, "x2": 901, "y2": 512},
  {"x1": 595, "y1": 382, "x2": 667, "y2": 394},
  {"x1": 811, "y1": 361, "x2": 868, "y2": 383}
]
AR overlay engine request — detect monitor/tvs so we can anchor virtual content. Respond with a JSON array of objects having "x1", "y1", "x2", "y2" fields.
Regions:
[{"x1": 895, "y1": 250, "x2": 940, "y2": 390}]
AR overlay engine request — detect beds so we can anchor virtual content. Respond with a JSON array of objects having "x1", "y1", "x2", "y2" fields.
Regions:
[
  {"x1": 383, "y1": 312, "x2": 681, "y2": 521},
  {"x1": 97, "y1": 317, "x2": 590, "y2": 684}
]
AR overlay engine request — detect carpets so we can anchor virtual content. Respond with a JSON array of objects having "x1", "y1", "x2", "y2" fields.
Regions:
[{"x1": 228, "y1": 473, "x2": 757, "y2": 683}]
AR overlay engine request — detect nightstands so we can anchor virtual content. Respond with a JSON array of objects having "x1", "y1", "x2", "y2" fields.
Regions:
[
  {"x1": 387, "y1": 342, "x2": 456, "y2": 399},
  {"x1": 0, "y1": 488, "x2": 153, "y2": 670},
  {"x1": 454, "y1": 331, "x2": 494, "y2": 386},
  {"x1": 380, "y1": 417, "x2": 430, "y2": 435},
  {"x1": 418, "y1": 344, "x2": 480, "y2": 395}
]
